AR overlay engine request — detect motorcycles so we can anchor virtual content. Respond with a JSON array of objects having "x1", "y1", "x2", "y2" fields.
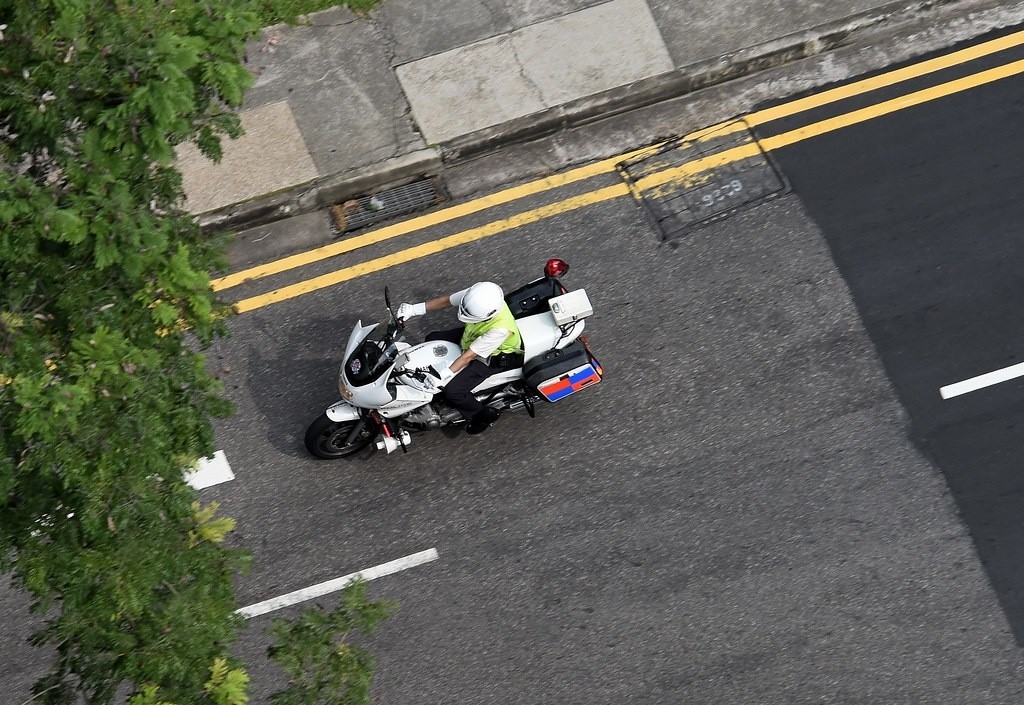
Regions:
[{"x1": 307, "y1": 257, "x2": 602, "y2": 460}]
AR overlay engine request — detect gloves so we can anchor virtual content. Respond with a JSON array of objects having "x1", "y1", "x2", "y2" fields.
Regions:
[
  {"x1": 396, "y1": 302, "x2": 427, "y2": 323},
  {"x1": 424, "y1": 366, "x2": 454, "y2": 390}
]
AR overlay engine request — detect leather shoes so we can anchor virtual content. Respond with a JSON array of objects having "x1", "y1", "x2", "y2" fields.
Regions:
[{"x1": 467, "y1": 406, "x2": 500, "y2": 435}]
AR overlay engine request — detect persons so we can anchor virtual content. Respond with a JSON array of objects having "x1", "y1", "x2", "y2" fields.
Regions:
[{"x1": 396, "y1": 281, "x2": 521, "y2": 435}]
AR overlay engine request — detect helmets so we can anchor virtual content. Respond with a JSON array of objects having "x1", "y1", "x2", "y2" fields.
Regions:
[{"x1": 458, "y1": 281, "x2": 504, "y2": 324}]
